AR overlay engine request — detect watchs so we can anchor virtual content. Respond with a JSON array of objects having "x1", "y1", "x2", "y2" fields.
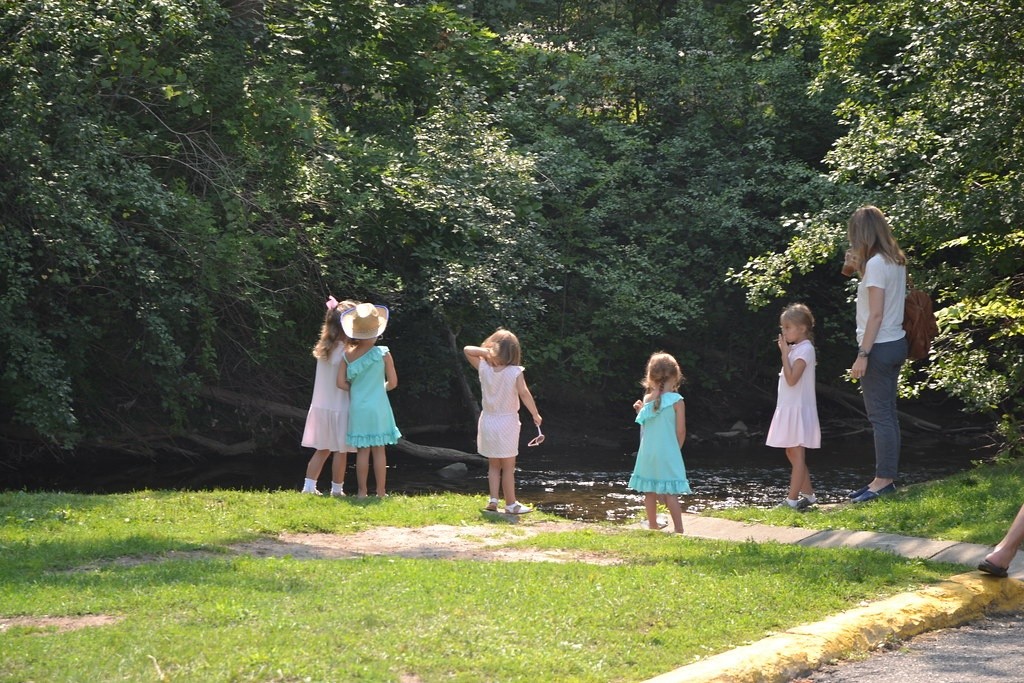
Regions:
[{"x1": 858, "y1": 348, "x2": 869, "y2": 357}]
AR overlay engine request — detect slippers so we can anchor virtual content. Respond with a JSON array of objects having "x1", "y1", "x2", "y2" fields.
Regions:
[
  {"x1": 772, "y1": 494, "x2": 818, "y2": 510},
  {"x1": 977, "y1": 560, "x2": 1010, "y2": 579}
]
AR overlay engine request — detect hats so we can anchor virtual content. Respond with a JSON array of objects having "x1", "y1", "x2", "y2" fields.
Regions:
[{"x1": 340, "y1": 303, "x2": 389, "y2": 340}]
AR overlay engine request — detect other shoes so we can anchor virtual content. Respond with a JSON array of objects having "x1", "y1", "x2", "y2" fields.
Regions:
[{"x1": 848, "y1": 482, "x2": 897, "y2": 503}]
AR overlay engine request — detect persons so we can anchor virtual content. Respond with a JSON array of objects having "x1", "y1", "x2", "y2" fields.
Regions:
[
  {"x1": 978, "y1": 504, "x2": 1024, "y2": 577},
  {"x1": 464, "y1": 329, "x2": 542, "y2": 514},
  {"x1": 766, "y1": 304, "x2": 821, "y2": 512},
  {"x1": 337, "y1": 303, "x2": 403, "y2": 498},
  {"x1": 847, "y1": 205, "x2": 908, "y2": 503},
  {"x1": 628, "y1": 351, "x2": 692, "y2": 534},
  {"x1": 301, "y1": 295, "x2": 360, "y2": 496}
]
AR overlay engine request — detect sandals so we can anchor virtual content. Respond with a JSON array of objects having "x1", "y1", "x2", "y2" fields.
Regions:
[
  {"x1": 484, "y1": 497, "x2": 498, "y2": 512},
  {"x1": 505, "y1": 501, "x2": 533, "y2": 514}
]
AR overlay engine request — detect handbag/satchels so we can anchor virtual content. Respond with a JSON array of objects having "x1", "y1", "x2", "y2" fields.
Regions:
[{"x1": 902, "y1": 279, "x2": 940, "y2": 362}]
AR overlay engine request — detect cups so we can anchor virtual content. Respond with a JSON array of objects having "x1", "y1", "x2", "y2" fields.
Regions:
[{"x1": 842, "y1": 249, "x2": 857, "y2": 278}]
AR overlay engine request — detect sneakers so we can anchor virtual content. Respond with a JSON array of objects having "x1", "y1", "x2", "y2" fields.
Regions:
[
  {"x1": 331, "y1": 491, "x2": 347, "y2": 499},
  {"x1": 301, "y1": 488, "x2": 323, "y2": 497}
]
